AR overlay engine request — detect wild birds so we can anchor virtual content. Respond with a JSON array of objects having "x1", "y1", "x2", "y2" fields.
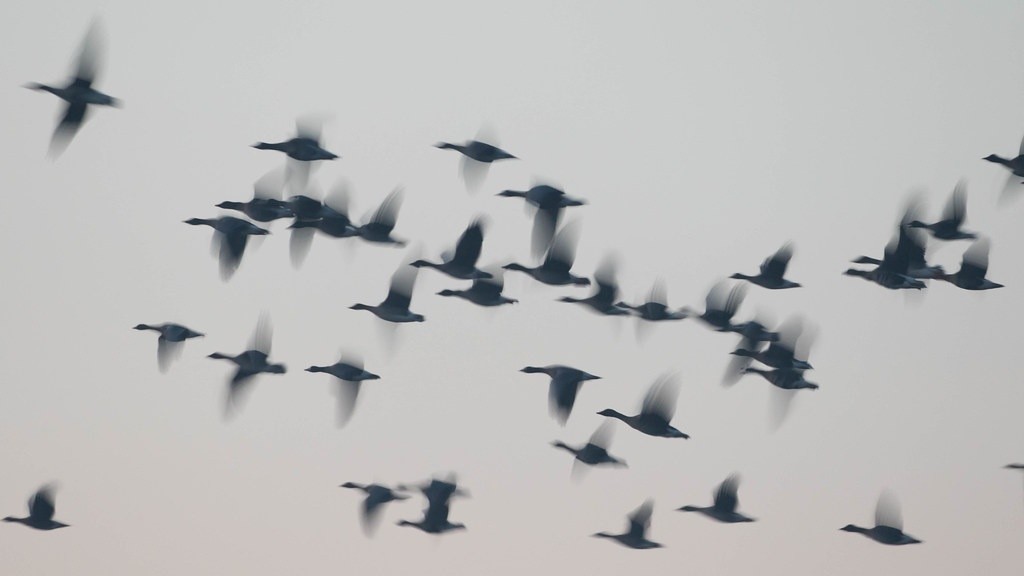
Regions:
[
  {"x1": 337, "y1": 470, "x2": 480, "y2": 539},
  {"x1": 130, "y1": 110, "x2": 1024, "y2": 482},
  {"x1": 837, "y1": 491, "x2": 922, "y2": 547},
  {"x1": 592, "y1": 500, "x2": 663, "y2": 549},
  {"x1": 0, "y1": 483, "x2": 72, "y2": 531},
  {"x1": 672, "y1": 475, "x2": 757, "y2": 524},
  {"x1": 23, "y1": 20, "x2": 129, "y2": 158}
]
